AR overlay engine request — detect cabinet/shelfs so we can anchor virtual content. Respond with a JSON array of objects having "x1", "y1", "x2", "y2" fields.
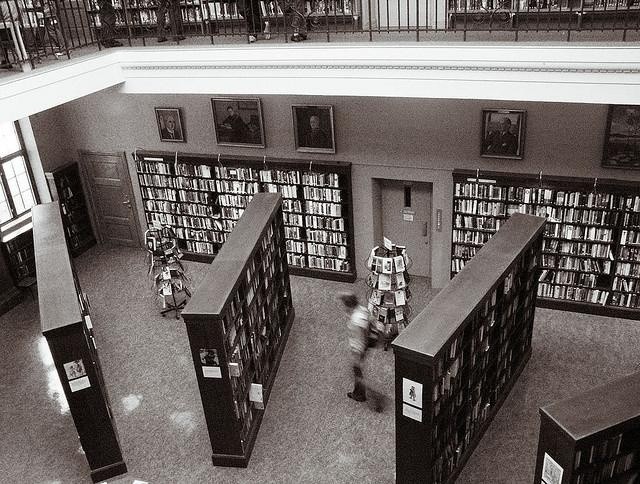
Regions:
[
  {"x1": 533, "y1": 370, "x2": 640, "y2": 483},
  {"x1": 181, "y1": 193, "x2": 294, "y2": 468},
  {"x1": 46, "y1": 162, "x2": 99, "y2": 258},
  {"x1": 390, "y1": 212, "x2": 547, "y2": 483},
  {"x1": 449, "y1": 169, "x2": 640, "y2": 320},
  {"x1": 130, "y1": 150, "x2": 357, "y2": 284},
  {"x1": 30, "y1": 200, "x2": 129, "y2": 482},
  {"x1": 3, "y1": 223, "x2": 37, "y2": 297}
]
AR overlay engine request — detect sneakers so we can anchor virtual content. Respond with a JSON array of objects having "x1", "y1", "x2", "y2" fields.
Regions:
[{"x1": 347, "y1": 392, "x2": 365, "y2": 402}]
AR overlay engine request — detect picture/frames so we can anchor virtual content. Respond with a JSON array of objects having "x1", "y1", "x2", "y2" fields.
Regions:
[
  {"x1": 154, "y1": 106, "x2": 185, "y2": 143},
  {"x1": 601, "y1": 105, "x2": 640, "y2": 170},
  {"x1": 479, "y1": 107, "x2": 527, "y2": 160},
  {"x1": 208, "y1": 97, "x2": 338, "y2": 155}
]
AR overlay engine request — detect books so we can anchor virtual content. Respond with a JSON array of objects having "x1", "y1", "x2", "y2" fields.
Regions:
[
  {"x1": 53, "y1": 164, "x2": 94, "y2": 251},
  {"x1": 364, "y1": 235, "x2": 414, "y2": 350},
  {"x1": 452, "y1": 179, "x2": 639, "y2": 308},
  {"x1": 434, "y1": 246, "x2": 537, "y2": 484},
  {"x1": 575, "y1": 429, "x2": 639, "y2": 484},
  {"x1": 138, "y1": 162, "x2": 350, "y2": 271},
  {"x1": 6, "y1": 230, "x2": 36, "y2": 282},
  {"x1": 222, "y1": 219, "x2": 290, "y2": 448}
]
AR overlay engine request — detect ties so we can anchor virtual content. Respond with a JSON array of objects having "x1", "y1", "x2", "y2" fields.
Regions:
[{"x1": 171, "y1": 133, "x2": 175, "y2": 139}]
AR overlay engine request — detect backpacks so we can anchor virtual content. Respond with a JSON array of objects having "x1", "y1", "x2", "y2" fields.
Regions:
[{"x1": 365, "y1": 322, "x2": 383, "y2": 349}]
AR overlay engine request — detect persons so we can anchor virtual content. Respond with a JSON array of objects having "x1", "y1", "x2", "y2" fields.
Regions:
[
  {"x1": 97, "y1": 0, "x2": 123, "y2": 47},
  {"x1": 32, "y1": 0, "x2": 69, "y2": 57},
  {"x1": 222, "y1": 106, "x2": 249, "y2": 143},
  {"x1": 487, "y1": 117, "x2": 518, "y2": 153},
  {"x1": 153, "y1": 1, "x2": 185, "y2": 42},
  {"x1": 0, "y1": 0, "x2": 22, "y2": 69},
  {"x1": 237, "y1": 1, "x2": 263, "y2": 41},
  {"x1": 279, "y1": 0, "x2": 306, "y2": 42},
  {"x1": 337, "y1": 290, "x2": 374, "y2": 402},
  {"x1": 305, "y1": 115, "x2": 328, "y2": 148},
  {"x1": 162, "y1": 116, "x2": 179, "y2": 141}
]
